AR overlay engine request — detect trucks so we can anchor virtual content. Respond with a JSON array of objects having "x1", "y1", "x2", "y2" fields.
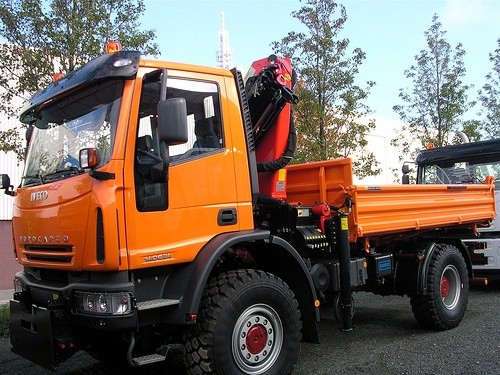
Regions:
[
  {"x1": 0, "y1": 41, "x2": 496, "y2": 375},
  {"x1": 402, "y1": 138, "x2": 500, "y2": 289}
]
